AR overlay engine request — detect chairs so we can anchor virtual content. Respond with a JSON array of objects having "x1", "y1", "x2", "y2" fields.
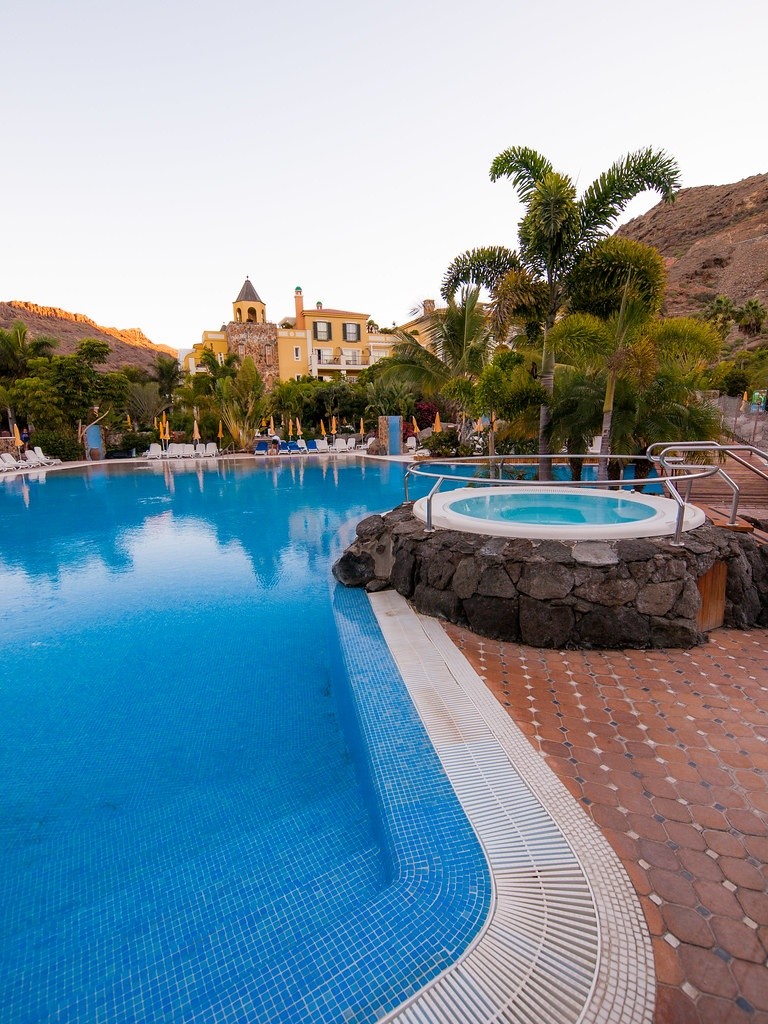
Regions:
[
  {"x1": 254, "y1": 439, "x2": 330, "y2": 455},
  {"x1": 147, "y1": 442, "x2": 219, "y2": 460},
  {"x1": 405, "y1": 437, "x2": 416, "y2": 452},
  {"x1": 0, "y1": 447, "x2": 62, "y2": 474},
  {"x1": 347, "y1": 438, "x2": 355, "y2": 450},
  {"x1": 336, "y1": 439, "x2": 350, "y2": 452},
  {"x1": 362, "y1": 438, "x2": 375, "y2": 449}
]
